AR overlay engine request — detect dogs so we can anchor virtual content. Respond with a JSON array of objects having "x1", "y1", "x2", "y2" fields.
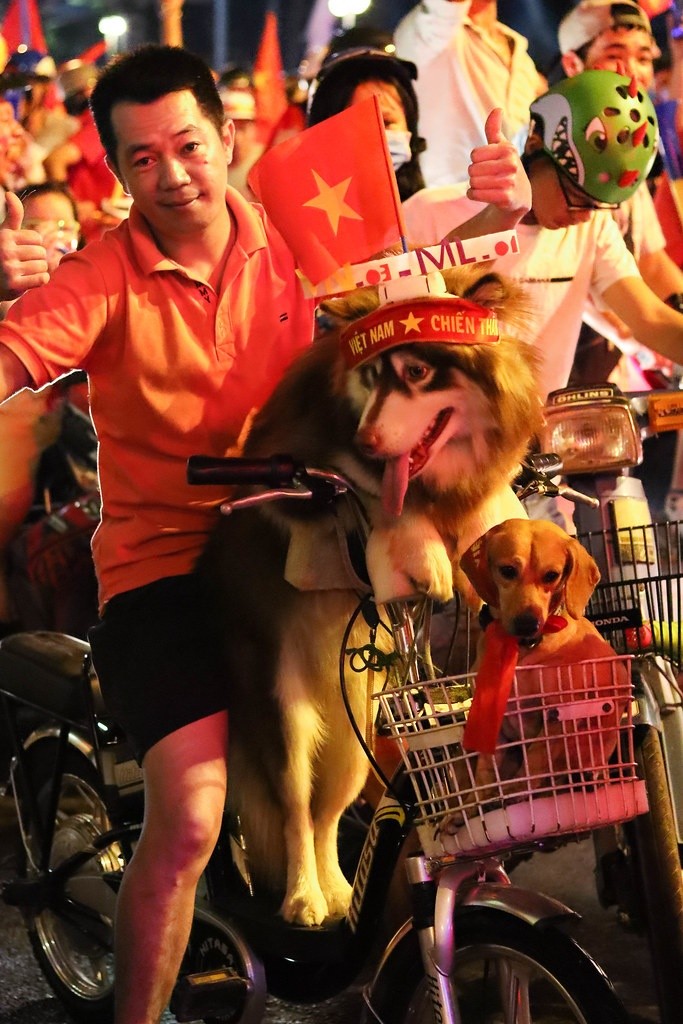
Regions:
[
  {"x1": 185, "y1": 266, "x2": 549, "y2": 928},
  {"x1": 438, "y1": 517, "x2": 632, "y2": 835}
]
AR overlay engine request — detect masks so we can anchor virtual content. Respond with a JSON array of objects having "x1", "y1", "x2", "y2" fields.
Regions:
[{"x1": 385, "y1": 129, "x2": 413, "y2": 173}]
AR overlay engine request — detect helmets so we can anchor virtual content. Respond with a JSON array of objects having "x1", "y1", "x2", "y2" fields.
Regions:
[{"x1": 528, "y1": 70, "x2": 660, "y2": 203}]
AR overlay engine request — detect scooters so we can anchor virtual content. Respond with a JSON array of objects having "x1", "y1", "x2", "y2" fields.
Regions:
[
  {"x1": 495, "y1": 379, "x2": 683, "y2": 1024},
  {"x1": 0, "y1": 452, "x2": 628, "y2": 1024}
]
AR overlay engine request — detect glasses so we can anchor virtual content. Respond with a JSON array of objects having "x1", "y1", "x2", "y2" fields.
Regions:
[
  {"x1": 554, "y1": 163, "x2": 621, "y2": 210},
  {"x1": 25, "y1": 220, "x2": 81, "y2": 239},
  {"x1": 320, "y1": 46, "x2": 397, "y2": 69}
]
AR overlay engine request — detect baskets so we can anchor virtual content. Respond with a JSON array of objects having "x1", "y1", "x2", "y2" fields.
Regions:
[
  {"x1": 374, "y1": 653, "x2": 647, "y2": 862},
  {"x1": 567, "y1": 519, "x2": 683, "y2": 670}
]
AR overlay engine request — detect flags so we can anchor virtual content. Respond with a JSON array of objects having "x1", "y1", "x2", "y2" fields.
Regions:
[
  {"x1": 0, "y1": 1, "x2": 47, "y2": 58},
  {"x1": 653, "y1": 171, "x2": 683, "y2": 269},
  {"x1": 252, "y1": 13, "x2": 287, "y2": 146},
  {"x1": 246, "y1": 96, "x2": 406, "y2": 287}
]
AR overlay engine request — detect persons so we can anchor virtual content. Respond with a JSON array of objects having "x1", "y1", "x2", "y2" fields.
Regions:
[{"x1": 0, "y1": 0, "x2": 683, "y2": 1024}]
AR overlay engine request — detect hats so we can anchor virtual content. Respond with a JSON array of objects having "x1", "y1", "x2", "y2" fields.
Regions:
[{"x1": 557, "y1": 0, "x2": 662, "y2": 60}]
